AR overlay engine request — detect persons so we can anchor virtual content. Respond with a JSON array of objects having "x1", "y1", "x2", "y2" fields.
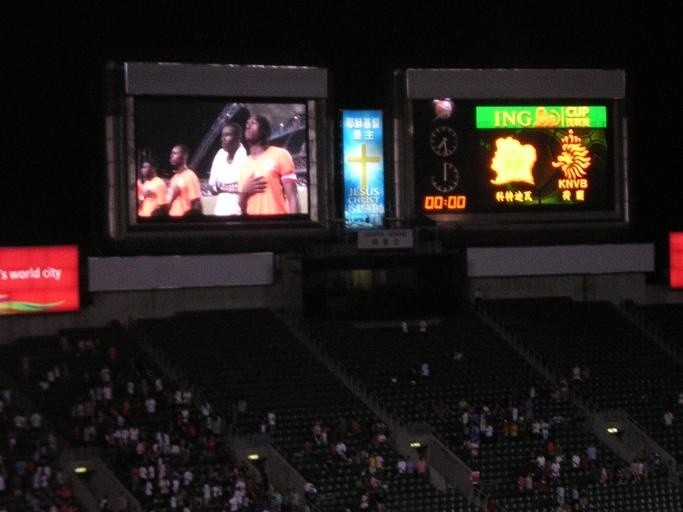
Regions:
[
  {"x1": 133, "y1": 110, "x2": 302, "y2": 221},
  {"x1": 1, "y1": 331, "x2": 321, "y2": 509},
  {"x1": 307, "y1": 318, "x2": 682, "y2": 509}
]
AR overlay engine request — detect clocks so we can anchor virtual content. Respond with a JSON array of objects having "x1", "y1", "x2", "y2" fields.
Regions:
[
  {"x1": 430, "y1": 127, "x2": 458, "y2": 156},
  {"x1": 431, "y1": 162, "x2": 459, "y2": 193}
]
